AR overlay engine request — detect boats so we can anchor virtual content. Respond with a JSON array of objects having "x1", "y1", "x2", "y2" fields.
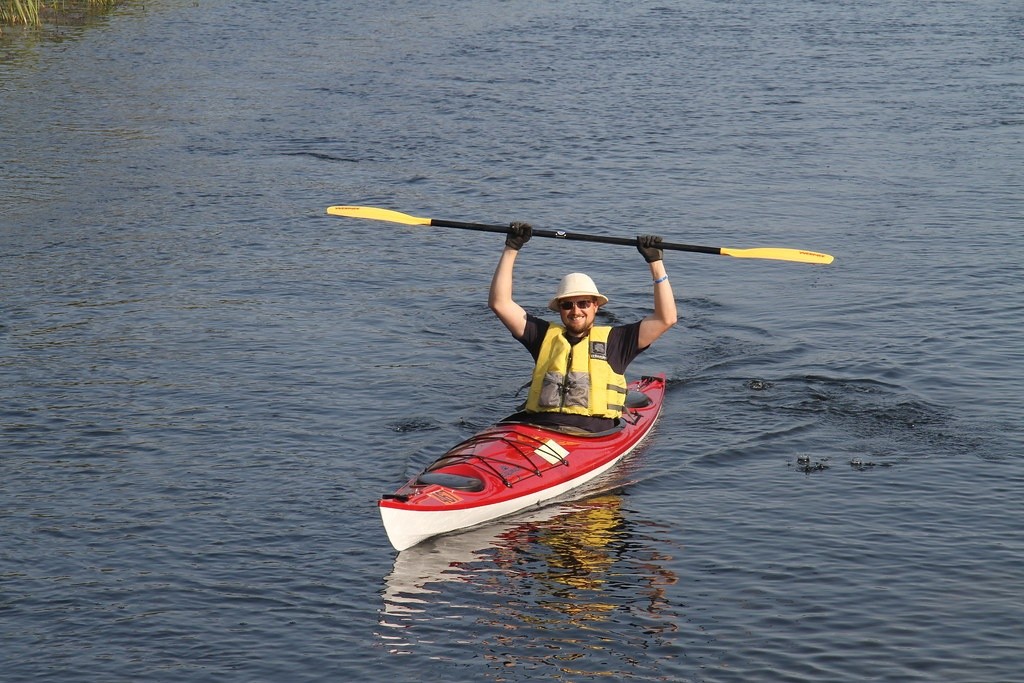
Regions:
[{"x1": 378, "y1": 372, "x2": 666, "y2": 552}]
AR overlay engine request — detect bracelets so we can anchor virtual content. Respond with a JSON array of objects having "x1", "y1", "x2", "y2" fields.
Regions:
[{"x1": 653, "y1": 275, "x2": 667, "y2": 284}]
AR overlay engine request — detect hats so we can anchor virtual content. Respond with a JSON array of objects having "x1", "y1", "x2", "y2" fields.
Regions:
[{"x1": 548, "y1": 273, "x2": 608, "y2": 312}]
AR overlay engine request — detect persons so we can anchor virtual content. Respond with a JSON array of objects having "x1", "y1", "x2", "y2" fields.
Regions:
[{"x1": 487, "y1": 222, "x2": 678, "y2": 433}]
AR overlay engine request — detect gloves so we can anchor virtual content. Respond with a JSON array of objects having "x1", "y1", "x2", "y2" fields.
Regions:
[
  {"x1": 637, "y1": 235, "x2": 663, "y2": 263},
  {"x1": 505, "y1": 221, "x2": 532, "y2": 250}
]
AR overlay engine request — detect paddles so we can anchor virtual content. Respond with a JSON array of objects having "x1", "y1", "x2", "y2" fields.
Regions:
[{"x1": 324, "y1": 203, "x2": 837, "y2": 267}]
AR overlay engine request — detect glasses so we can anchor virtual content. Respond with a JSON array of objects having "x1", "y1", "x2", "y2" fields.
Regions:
[{"x1": 559, "y1": 300, "x2": 595, "y2": 310}]
